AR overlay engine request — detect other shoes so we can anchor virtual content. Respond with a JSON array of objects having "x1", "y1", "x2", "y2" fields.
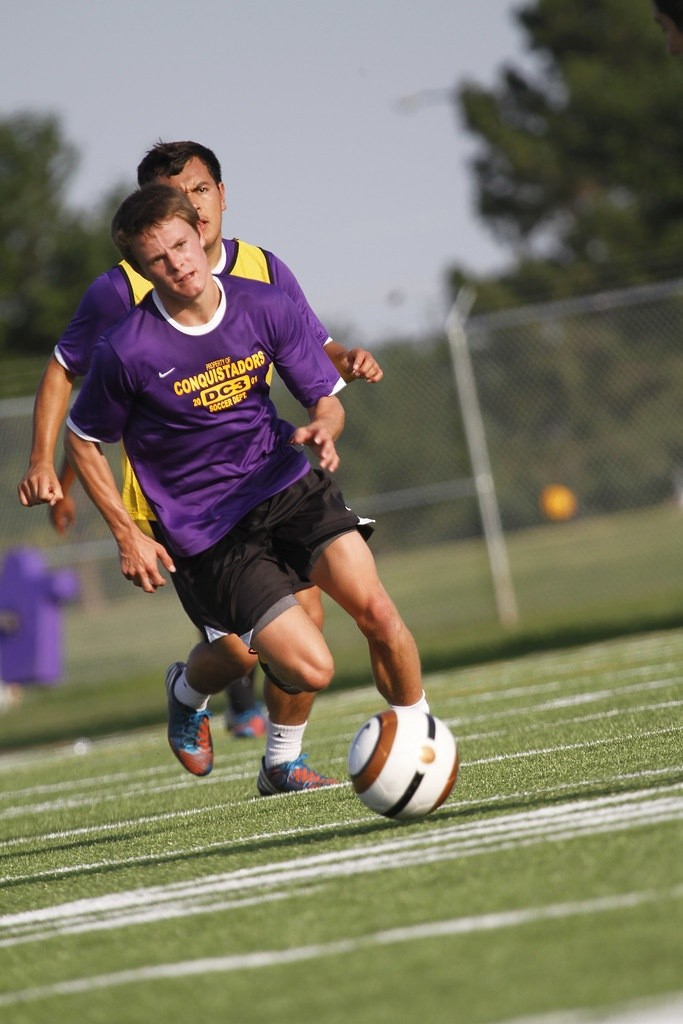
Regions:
[{"x1": 226, "y1": 692, "x2": 263, "y2": 738}]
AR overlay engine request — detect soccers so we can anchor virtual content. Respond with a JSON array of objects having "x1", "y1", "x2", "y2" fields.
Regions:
[{"x1": 348, "y1": 708, "x2": 460, "y2": 822}]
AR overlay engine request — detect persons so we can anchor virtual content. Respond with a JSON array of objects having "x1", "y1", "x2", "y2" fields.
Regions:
[
  {"x1": 65, "y1": 184, "x2": 431, "y2": 714},
  {"x1": 18, "y1": 137, "x2": 384, "y2": 794}
]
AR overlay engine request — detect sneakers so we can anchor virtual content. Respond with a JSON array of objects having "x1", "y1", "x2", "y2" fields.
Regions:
[
  {"x1": 256, "y1": 753, "x2": 339, "y2": 797},
  {"x1": 164, "y1": 662, "x2": 214, "y2": 778}
]
